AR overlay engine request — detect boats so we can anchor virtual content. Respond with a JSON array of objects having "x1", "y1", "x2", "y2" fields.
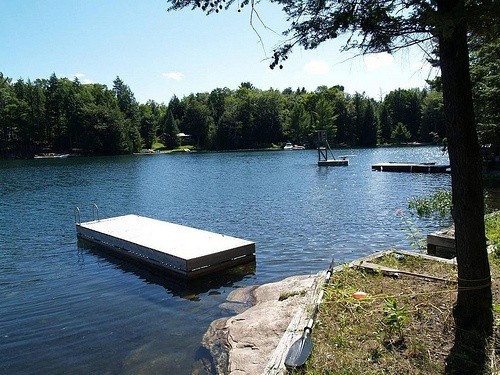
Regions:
[
  {"x1": 33, "y1": 152, "x2": 67, "y2": 158},
  {"x1": 283, "y1": 143, "x2": 306, "y2": 150}
]
